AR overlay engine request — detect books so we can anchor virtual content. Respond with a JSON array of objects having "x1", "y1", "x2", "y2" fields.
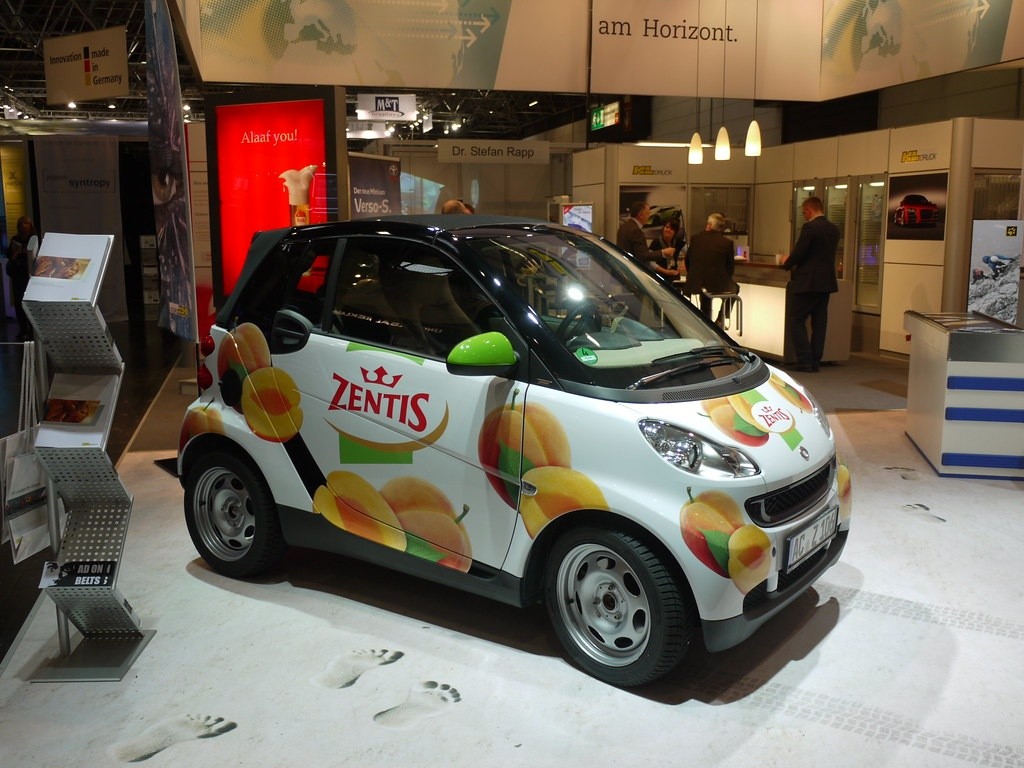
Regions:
[{"x1": 12, "y1": 240, "x2": 22, "y2": 254}]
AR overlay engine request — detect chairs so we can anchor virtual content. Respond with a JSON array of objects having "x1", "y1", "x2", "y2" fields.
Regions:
[{"x1": 389, "y1": 256, "x2": 484, "y2": 357}]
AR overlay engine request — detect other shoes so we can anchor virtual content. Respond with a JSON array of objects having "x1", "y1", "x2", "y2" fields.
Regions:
[
  {"x1": 16, "y1": 331, "x2": 33, "y2": 341},
  {"x1": 715, "y1": 321, "x2": 728, "y2": 330},
  {"x1": 783, "y1": 358, "x2": 812, "y2": 372},
  {"x1": 811, "y1": 359, "x2": 820, "y2": 372}
]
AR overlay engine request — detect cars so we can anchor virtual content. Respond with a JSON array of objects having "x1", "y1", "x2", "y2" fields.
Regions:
[
  {"x1": 175, "y1": 215, "x2": 854, "y2": 690},
  {"x1": 893, "y1": 195, "x2": 939, "y2": 226}
]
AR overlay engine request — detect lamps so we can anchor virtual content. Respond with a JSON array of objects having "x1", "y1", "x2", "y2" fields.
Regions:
[
  {"x1": 687, "y1": 1, "x2": 703, "y2": 164},
  {"x1": 745, "y1": 0, "x2": 762, "y2": 157},
  {"x1": 715, "y1": 1, "x2": 731, "y2": 160}
]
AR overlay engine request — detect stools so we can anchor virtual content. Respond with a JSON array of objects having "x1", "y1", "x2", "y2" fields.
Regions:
[{"x1": 701, "y1": 287, "x2": 743, "y2": 337}]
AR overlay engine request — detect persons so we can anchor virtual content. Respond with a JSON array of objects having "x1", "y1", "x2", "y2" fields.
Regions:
[
  {"x1": 778, "y1": 197, "x2": 840, "y2": 372},
  {"x1": 684, "y1": 212, "x2": 740, "y2": 330},
  {"x1": 441, "y1": 199, "x2": 478, "y2": 307},
  {"x1": 649, "y1": 219, "x2": 686, "y2": 284},
  {"x1": 616, "y1": 201, "x2": 675, "y2": 321},
  {"x1": 6, "y1": 217, "x2": 41, "y2": 341}
]
